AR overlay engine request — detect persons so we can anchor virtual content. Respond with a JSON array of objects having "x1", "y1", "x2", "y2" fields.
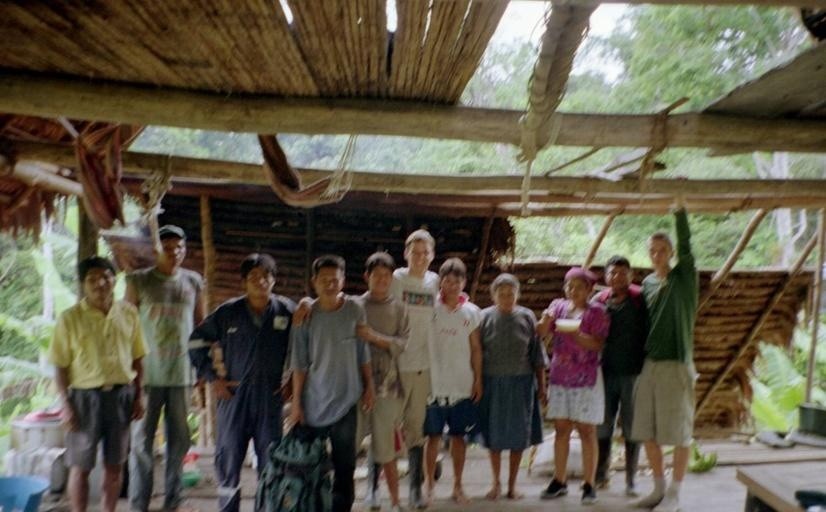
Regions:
[
  {"x1": 589, "y1": 254, "x2": 651, "y2": 497},
  {"x1": 536, "y1": 267, "x2": 611, "y2": 504},
  {"x1": 630, "y1": 192, "x2": 700, "y2": 512},
  {"x1": 373, "y1": 229, "x2": 442, "y2": 512},
  {"x1": 289, "y1": 252, "x2": 413, "y2": 512},
  {"x1": 49, "y1": 258, "x2": 150, "y2": 512},
  {"x1": 122, "y1": 225, "x2": 205, "y2": 512},
  {"x1": 422, "y1": 258, "x2": 481, "y2": 508},
  {"x1": 465, "y1": 274, "x2": 550, "y2": 501},
  {"x1": 188, "y1": 254, "x2": 298, "y2": 512}
]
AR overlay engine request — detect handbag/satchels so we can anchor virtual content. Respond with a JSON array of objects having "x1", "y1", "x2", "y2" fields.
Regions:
[{"x1": 254, "y1": 427, "x2": 332, "y2": 512}]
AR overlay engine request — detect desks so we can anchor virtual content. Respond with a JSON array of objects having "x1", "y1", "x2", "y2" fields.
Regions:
[{"x1": 736, "y1": 464, "x2": 826, "y2": 512}]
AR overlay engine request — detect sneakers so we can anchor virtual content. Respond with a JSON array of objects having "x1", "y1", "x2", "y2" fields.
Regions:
[
  {"x1": 651, "y1": 495, "x2": 681, "y2": 512},
  {"x1": 630, "y1": 492, "x2": 664, "y2": 510},
  {"x1": 625, "y1": 473, "x2": 639, "y2": 495},
  {"x1": 540, "y1": 478, "x2": 568, "y2": 499},
  {"x1": 592, "y1": 478, "x2": 610, "y2": 491},
  {"x1": 582, "y1": 479, "x2": 597, "y2": 504}
]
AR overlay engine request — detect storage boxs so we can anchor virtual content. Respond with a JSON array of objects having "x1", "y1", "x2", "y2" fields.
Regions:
[{"x1": 4, "y1": 419, "x2": 66, "y2": 489}]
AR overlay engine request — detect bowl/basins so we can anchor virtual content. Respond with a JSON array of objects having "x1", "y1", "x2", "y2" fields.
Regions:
[
  {"x1": 183, "y1": 472, "x2": 202, "y2": 487},
  {"x1": 554, "y1": 317, "x2": 583, "y2": 332}
]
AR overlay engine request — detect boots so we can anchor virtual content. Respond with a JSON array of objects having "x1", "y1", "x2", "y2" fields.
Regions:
[
  {"x1": 408, "y1": 443, "x2": 428, "y2": 511},
  {"x1": 366, "y1": 461, "x2": 383, "y2": 509}
]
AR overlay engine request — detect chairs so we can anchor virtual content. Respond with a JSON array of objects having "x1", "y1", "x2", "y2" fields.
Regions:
[{"x1": 0, "y1": 475, "x2": 51, "y2": 512}]
